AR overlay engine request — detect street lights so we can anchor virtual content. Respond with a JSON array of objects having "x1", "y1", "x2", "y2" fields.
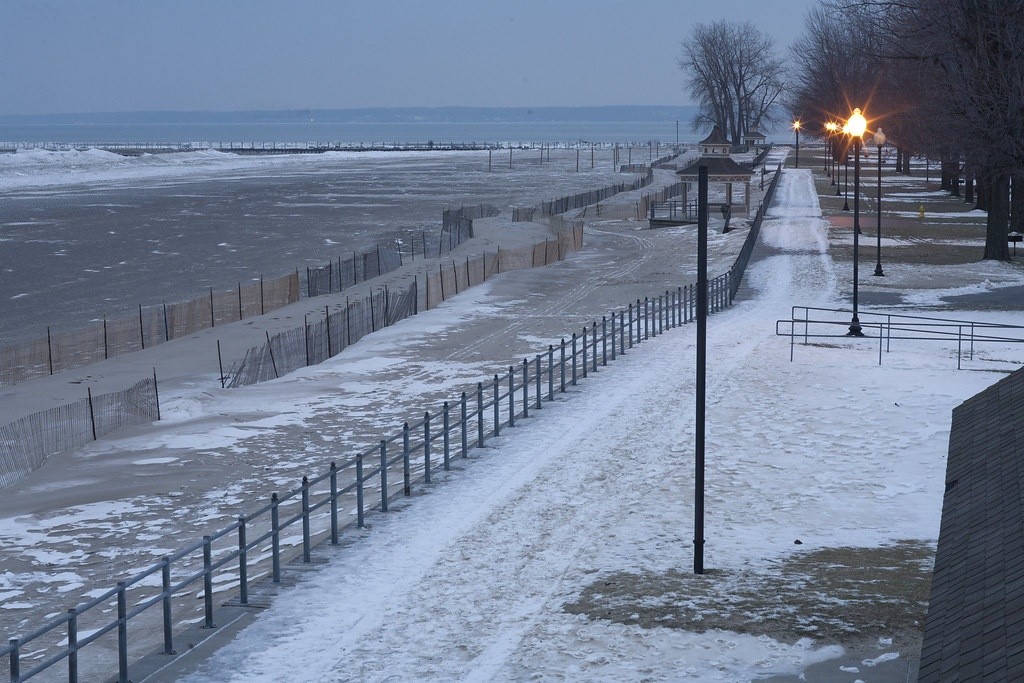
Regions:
[
  {"x1": 792, "y1": 121, "x2": 801, "y2": 168},
  {"x1": 823, "y1": 106, "x2": 887, "y2": 338}
]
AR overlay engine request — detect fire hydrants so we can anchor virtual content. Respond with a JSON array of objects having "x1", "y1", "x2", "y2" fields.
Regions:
[{"x1": 918, "y1": 205, "x2": 925, "y2": 218}]
